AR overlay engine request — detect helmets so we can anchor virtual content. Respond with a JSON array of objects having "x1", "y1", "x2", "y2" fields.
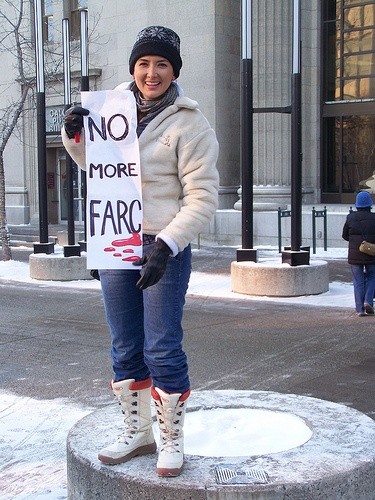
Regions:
[{"x1": 356, "y1": 192, "x2": 373, "y2": 208}]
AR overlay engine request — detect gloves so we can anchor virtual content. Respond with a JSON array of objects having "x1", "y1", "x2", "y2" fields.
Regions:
[
  {"x1": 64, "y1": 105, "x2": 89, "y2": 139},
  {"x1": 132, "y1": 238, "x2": 171, "y2": 291}
]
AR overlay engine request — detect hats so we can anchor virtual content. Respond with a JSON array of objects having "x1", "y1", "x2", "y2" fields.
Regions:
[{"x1": 129, "y1": 26, "x2": 182, "y2": 79}]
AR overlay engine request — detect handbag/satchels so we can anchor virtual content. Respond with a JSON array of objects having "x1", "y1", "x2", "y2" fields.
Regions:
[{"x1": 359, "y1": 241, "x2": 375, "y2": 256}]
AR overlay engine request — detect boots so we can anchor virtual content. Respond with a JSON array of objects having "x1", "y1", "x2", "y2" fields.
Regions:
[
  {"x1": 151, "y1": 384, "x2": 191, "y2": 477},
  {"x1": 98, "y1": 376, "x2": 156, "y2": 465}
]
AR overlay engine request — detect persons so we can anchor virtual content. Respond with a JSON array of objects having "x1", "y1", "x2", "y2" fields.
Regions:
[
  {"x1": 60, "y1": 24, "x2": 221, "y2": 478},
  {"x1": 341, "y1": 191, "x2": 375, "y2": 317}
]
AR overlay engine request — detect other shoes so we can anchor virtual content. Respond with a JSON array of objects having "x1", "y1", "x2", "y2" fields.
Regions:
[
  {"x1": 364, "y1": 303, "x2": 374, "y2": 315},
  {"x1": 358, "y1": 313, "x2": 367, "y2": 316}
]
